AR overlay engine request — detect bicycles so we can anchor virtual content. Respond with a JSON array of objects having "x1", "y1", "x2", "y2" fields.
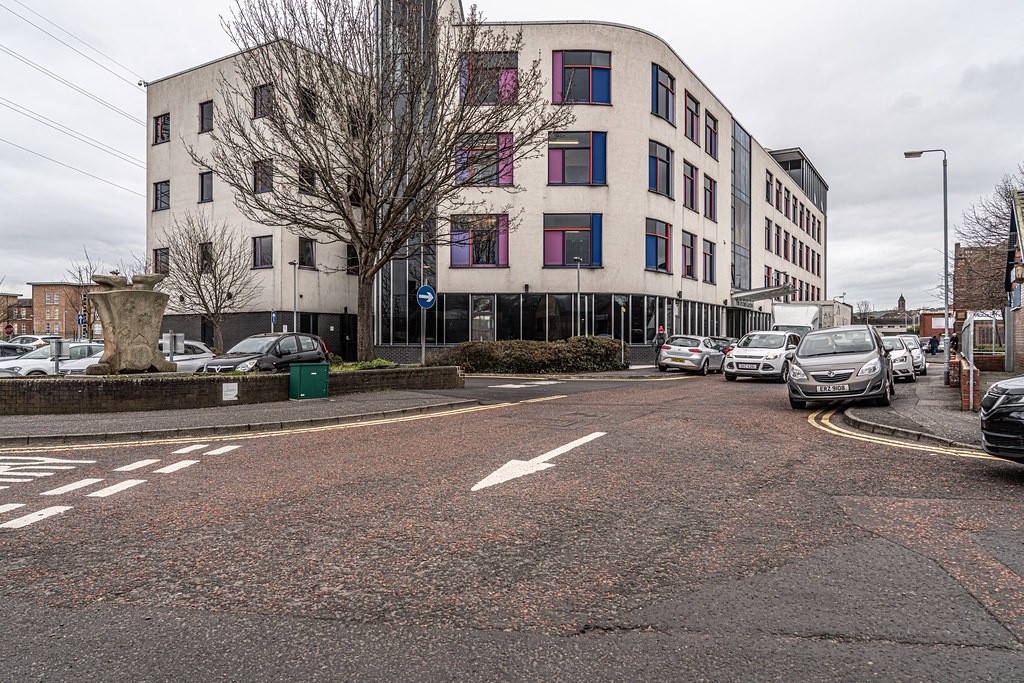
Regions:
[{"x1": 653, "y1": 343, "x2": 663, "y2": 369}]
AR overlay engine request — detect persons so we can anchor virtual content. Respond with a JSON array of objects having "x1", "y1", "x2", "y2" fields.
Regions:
[
  {"x1": 651, "y1": 326, "x2": 669, "y2": 353},
  {"x1": 950, "y1": 333, "x2": 959, "y2": 355},
  {"x1": 929, "y1": 335, "x2": 939, "y2": 355}
]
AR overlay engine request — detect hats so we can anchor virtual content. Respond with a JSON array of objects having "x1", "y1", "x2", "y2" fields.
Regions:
[
  {"x1": 658, "y1": 326, "x2": 663, "y2": 331},
  {"x1": 952, "y1": 333, "x2": 957, "y2": 335}
]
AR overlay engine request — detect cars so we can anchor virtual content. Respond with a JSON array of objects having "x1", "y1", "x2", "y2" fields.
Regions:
[
  {"x1": 0, "y1": 334, "x2": 107, "y2": 378},
  {"x1": 979, "y1": 377, "x2": 1024, "y2": 463},
  {"x1": 56, "y1": 340, "x2": 218, "y2": 375},
  {"x1": 788, "y1": 325, "x2": 895, "y2": 412},
  {"x1": 204, "y1": 332, "x2": 335, "y2": 374},
  {"x1": 711, "y1": 325, "x2": 814, "y2": 381},
  {"x1": 658, "y1": 335, "x2": 724, "y2": 376},
  {"x1": 883, "y1": 334, "x2": 945, "y2": 380}
]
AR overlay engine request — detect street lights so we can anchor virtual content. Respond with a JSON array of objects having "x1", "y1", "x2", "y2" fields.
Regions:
[
  {"x1": 289, "y1": 260, "x2": 299, "y2": 331},
  {"x1": 903, "y1": 149, "x2": 948, "y2": 387},
  {"x1": 573, "y1": 257, "x2": 582, "y2": 338}
]
